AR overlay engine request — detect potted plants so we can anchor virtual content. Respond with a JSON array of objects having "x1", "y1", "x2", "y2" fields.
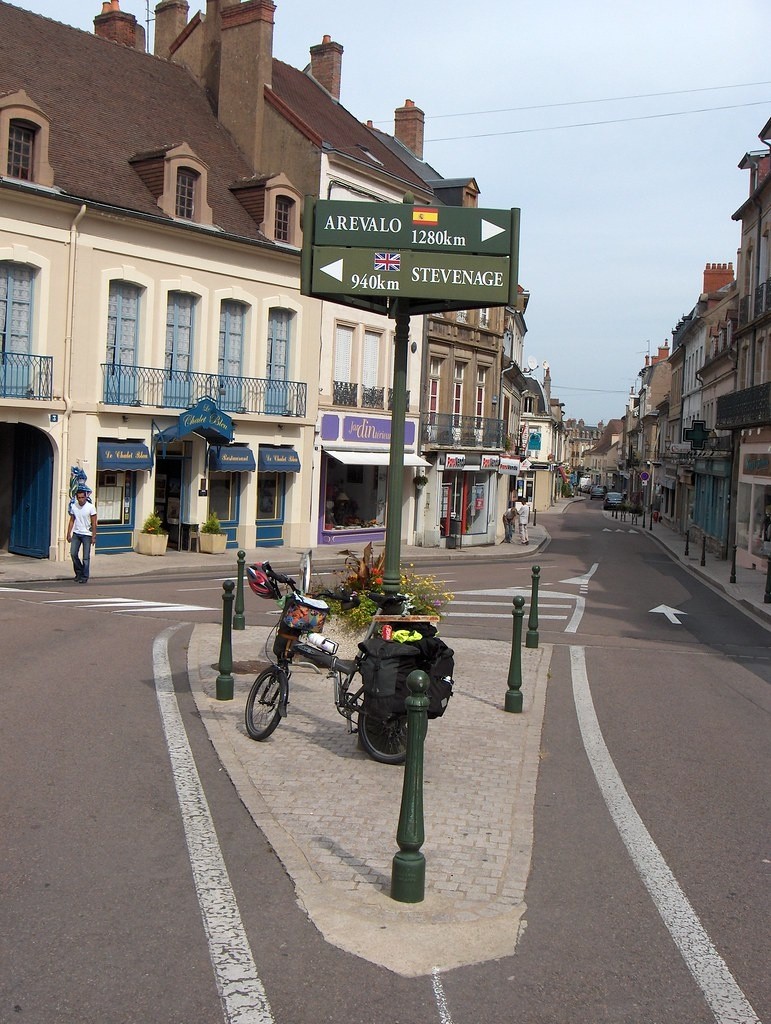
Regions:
[
  {"x1": 413, "y1": 475, "x2": 428, "y2": 489},
  {"x1": 200, "y1": 511, "x2": 228, "y2": 554},
  {"x1": 325, "y1": 519, "x2": 332, "y2": 529}
]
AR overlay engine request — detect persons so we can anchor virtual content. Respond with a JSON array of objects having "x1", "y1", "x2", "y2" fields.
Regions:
[
  {"x1": 67, "y1": 489, "x2": 97, "y2": 584},
  {"x1": 502, "y1": 507, "x2": 517, "y2": 543},
  {"x1": 516, "y1": 498, "x2": 529, "y2": 544}
]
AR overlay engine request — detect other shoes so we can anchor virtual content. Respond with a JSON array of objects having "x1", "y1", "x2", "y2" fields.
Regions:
[
  {"x1": 74, "y1": 574, "x2": 88, "y2": 584},
  {"x1": 509, "y1": 539, "x2": 516, "y2": 544},
  {"x1": 521, "y1": 540, "x2": 529, "y2": 544}
]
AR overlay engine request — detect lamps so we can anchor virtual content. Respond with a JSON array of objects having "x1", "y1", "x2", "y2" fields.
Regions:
[
  {"x1": 336, "y1": 493, "x2": 349, "y2": 506},
  {"x1": 219, "y1": 384, "x2": 226, "y2": 395}
]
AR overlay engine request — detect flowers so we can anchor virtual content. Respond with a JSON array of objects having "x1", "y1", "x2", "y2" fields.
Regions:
[
  {"x1": 141, "y1": 510, "x2": 170, "y2": 537},
  {"x1": 310, "y1": 543, "x2": 455, "y2": 633}
]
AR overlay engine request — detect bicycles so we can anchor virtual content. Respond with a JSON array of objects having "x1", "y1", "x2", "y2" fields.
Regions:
[{"x1": 244, "y1": 569, "x2": 440, "y2": 764}]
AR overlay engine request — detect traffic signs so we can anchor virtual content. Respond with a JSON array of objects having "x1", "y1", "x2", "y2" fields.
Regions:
[{"x1": 299, "y1": 193, "x2": 520, "y2": 315}]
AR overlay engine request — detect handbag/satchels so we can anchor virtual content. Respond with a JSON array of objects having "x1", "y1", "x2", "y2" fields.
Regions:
[
  {"x1": 281, "y1": 594, "x2": 329, "y2": 633},
  {"x1": 412, "y1": 633, "x2": 456, "y2": 719},
  {"x1": 358, "y1": 637, "x2": 419, "y2": 719}
]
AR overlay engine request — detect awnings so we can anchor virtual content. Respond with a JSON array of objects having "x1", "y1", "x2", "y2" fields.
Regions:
[
  {"x1": 258, "y1": 448, "x2": 301, "y2": 474},
  {"x1": 156, "y1": 398, "x2": 234, "y2": 444},
  {"x1": 210, "y1": 447, "x2": 256, "y2": 473},
  {"x1": 322, "y1": 444, "x2": 433, "y2": 466},
  {"x1": 97, "y1": 442, "x2": 153, "y2": 473}
]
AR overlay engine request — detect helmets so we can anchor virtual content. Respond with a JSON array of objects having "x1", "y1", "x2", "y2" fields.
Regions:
[{"x1": 246, "y1": 561, "x2": 277, "y2": 601}]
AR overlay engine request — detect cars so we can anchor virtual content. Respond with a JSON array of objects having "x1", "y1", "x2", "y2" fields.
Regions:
[
  {"x1": 603, "y1": 492, "x2": 625, "y2": 510},
  {"x1": 581, "y1": 484, "x2": 607, "y2": 493},
  {"x1": 590, "y1": 488, "x2": 605, "y2": 500}
]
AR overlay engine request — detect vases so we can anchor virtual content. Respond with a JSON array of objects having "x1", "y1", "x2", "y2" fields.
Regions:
[
  {"x1": 138, "y1": 533, "x2": 169, "y2": 555},
  {"x1": 317, "y1": 616, "x2": 374, "y2": 660}
]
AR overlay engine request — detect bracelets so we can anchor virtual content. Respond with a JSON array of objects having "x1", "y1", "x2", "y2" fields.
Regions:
[{"x1": 92, "y1": 536, "x2": 96, "y2": 538}]
